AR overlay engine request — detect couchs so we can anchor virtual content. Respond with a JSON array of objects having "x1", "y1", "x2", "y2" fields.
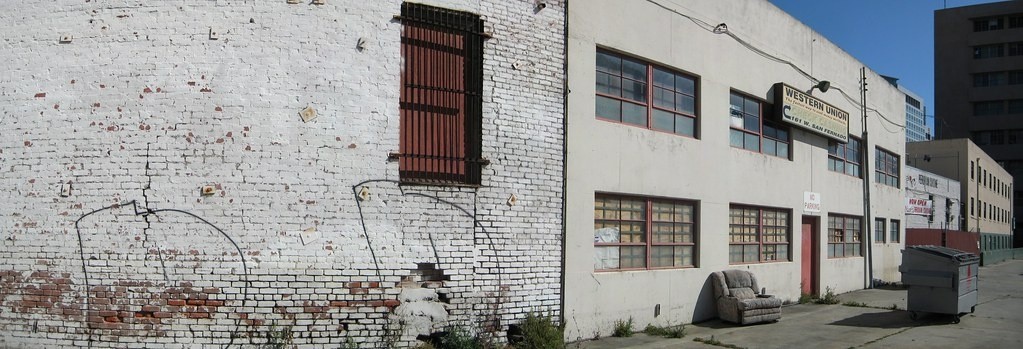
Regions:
[{"x1": 712, "y1": 269, "x2": 782, "y2": 327}]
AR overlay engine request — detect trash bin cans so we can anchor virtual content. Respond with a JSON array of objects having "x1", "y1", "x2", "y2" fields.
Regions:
[{"x1": 898, "y1": 244, "x2": 979, "y2": 324}]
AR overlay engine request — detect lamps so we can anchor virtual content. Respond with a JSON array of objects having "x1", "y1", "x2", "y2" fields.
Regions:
[{"x1": 805, "y1": 80, "x2": 830, "y2": 96}]
